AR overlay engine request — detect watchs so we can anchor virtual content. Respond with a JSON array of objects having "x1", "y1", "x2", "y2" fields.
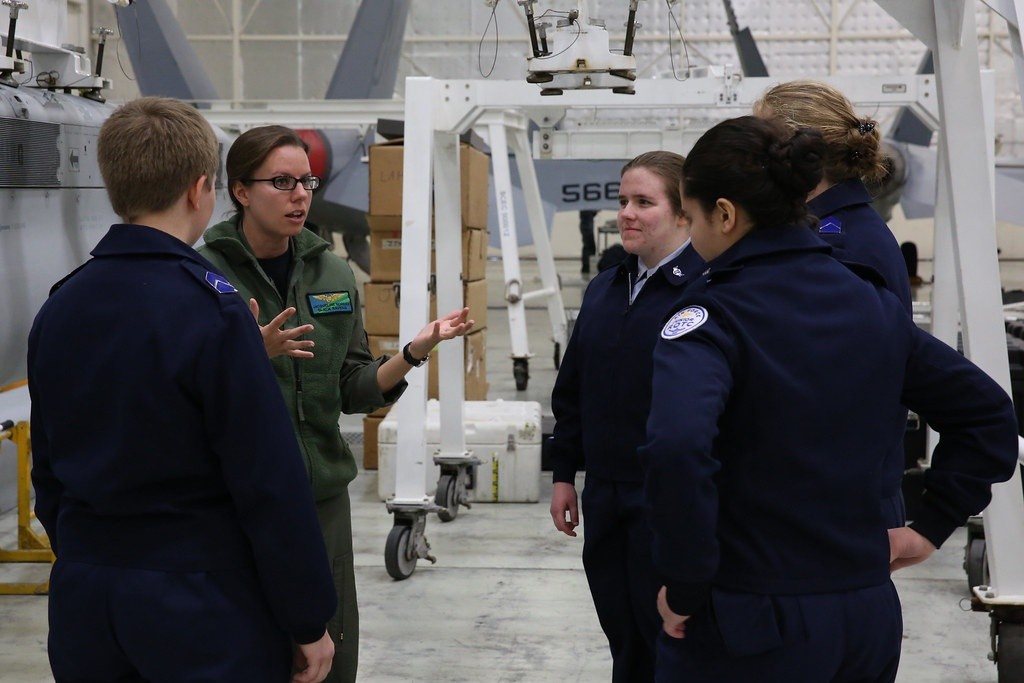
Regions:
[{"x1": 403, "y1": 342, "x2": 430, "y2": 368}]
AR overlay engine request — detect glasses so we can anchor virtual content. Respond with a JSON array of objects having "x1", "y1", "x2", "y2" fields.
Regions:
[{"x1": 241, "y1": 175, "x2": 322, "y2": 191}]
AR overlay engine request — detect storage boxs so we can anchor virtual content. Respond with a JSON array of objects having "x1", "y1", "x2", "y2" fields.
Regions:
[
  {"x1": 362, "y1": 416, "x2": 388, "y2": 470},
  {"x1": 367, "y1": 327, "x2": 486, "y2": 417},
  {"x1": 367, "y1": 136, "x2": 490, "y2": 230},
  {"x1": 365, "y1": 214, "x2": 488, "y2": 282},
  {"x1": 377, "y1": 399, "x2": 542, "y2": 504},
  {"x1": 377, "y1": 117, "x2": 492, "y2": 155},
  {"x1": 363, "y1": 277, "x2": 487, "y2": 336}
]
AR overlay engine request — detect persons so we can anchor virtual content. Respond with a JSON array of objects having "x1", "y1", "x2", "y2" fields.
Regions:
[
  {"x1": 27, "y1": 95, "x2": 335, "y2": 683},
  {"x1": 192, "y1": 125, "x2": 475, "y2": 683},
  {"x1": 647, "y1": 116, "x2": 1018, "y2": 683},
  {"x1": 550, "y1": 151, "x2": 707, "y2": 683},
  {"x1": 753, "y1": 81, "x2": 913, "y2": 530}
]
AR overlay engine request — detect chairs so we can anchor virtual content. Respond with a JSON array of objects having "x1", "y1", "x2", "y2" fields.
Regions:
[{"x1": 597, "y1": 220, "x2": 620, "y2": 255}]
